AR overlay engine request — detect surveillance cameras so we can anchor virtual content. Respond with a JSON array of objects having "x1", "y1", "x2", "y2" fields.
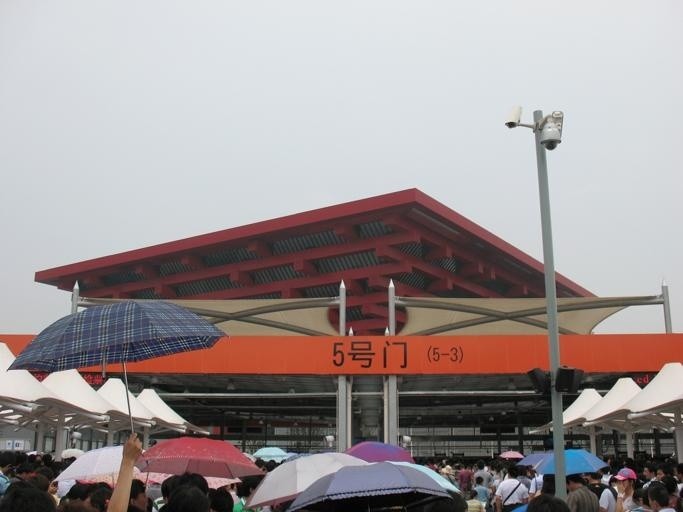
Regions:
[
  {"x1": 540, "y1": 123, "x2": 561, "y2": 150},
  {"x1": 505, "y1": 105, "x2": 521, "y2": 128}
]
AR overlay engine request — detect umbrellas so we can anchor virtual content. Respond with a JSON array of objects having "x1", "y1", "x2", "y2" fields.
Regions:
[{"x1": 7, "y1": 299, "x2": 230, "y2": 433}]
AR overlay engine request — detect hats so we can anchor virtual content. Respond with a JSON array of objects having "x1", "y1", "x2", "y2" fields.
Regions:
[
  {"x1": 614, "y1": 468, "x2": 637, "y2": 481},
  {"x1": 442, "y1": 465, "x2": 455, "y2": 476}
]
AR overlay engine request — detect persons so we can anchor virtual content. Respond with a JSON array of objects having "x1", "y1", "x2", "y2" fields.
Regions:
[
  {"x1": 423, "y1": 456, "x2": 683, "y2": 512},
  {"x1": 1, "y1": 433, "x2": 288, "y2": 512}
]
